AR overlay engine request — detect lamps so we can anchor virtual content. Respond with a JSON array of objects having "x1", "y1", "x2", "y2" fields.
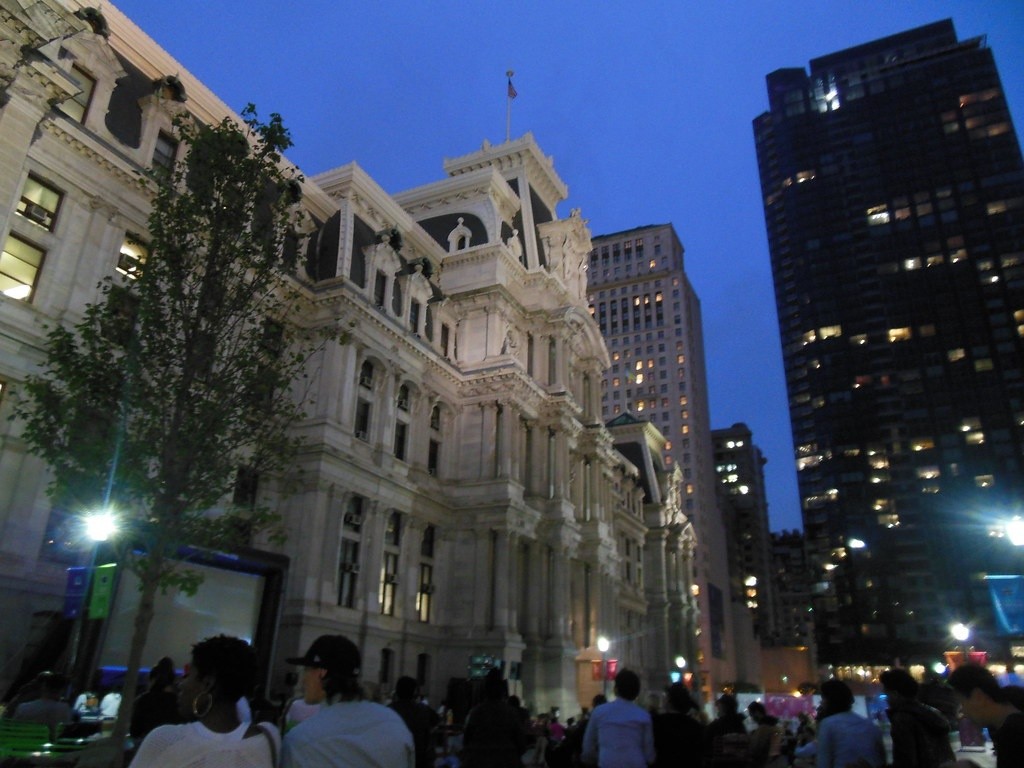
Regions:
[{"x1": 136, "y1": 76, "x2": 188, "y2": 108}]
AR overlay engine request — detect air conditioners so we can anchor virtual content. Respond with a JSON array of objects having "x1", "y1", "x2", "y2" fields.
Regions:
[
  {"x1": 428, "y1": 467, "x2": 435, "y2": 477},
  {"x1": 356, "y1": 430, "x2": 368, "y2": 442},
  {"x1": 347, "y1": 562, "x2": 361, "y2": 574},
  {"x1": 386, "y1": 574, "x2": 401, "y2": 586},
  {"x1": 398, "y1": 399, "x2": 410, "y2": 410},
  {"x1": 361, "y1": 376, "x2": 375, "y2": 388},
  {"x1": 413, "y1": 333, "x2": 421, "y2": 340},
  {"x1": 378, "y1": 305, "x2": 386, "y2": 313},
  {"x1": 344, "y1": 512, "x2": 363, "y2": 526},
  {"x1": 422, "y1": 583, "x2": 436, "y2": 595}
]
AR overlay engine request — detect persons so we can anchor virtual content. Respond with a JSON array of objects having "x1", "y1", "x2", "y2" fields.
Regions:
[
  {"x1": 127, "y1": 635, "x2": 283, "y2": 768},
  {"x1": 581, "y1": 668, "x2": 656, "y2": 768},
  {"x1": 280, "y1": 634, "x2": 415, "y2": 768},
  {"x1": 458, "y1": 668, "x2": 779, "y2": 768},
  {"x1": 387, "y1": 677, "x2": 439, "y2": 768},
  {"x1": 130, "y1": 657, "x2": 193, "y2": 745},
  {"x1": 782, "y1": 664, "x2": 1024, "y2": 768},
  {"x1": 285, "y1": 699, "x2": 320, "y2": 729}
]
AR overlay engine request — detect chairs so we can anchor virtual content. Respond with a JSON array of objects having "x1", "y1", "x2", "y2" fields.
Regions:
[
  {"x1": 0, "y1": 717, "x2": 143, "y2": 768},
  {"x1": 710, "y1": 735, "x2": 758, "y2": 768}
]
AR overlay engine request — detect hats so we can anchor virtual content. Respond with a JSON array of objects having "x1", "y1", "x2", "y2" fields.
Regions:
[{"x1": 285, "y1": 634, "x2": 363, "y2": 675}]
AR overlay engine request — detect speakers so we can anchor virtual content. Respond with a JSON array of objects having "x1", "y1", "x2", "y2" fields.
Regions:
[{"x1": 511, "y1": 661, "x2": 521, "y2": 680}]
